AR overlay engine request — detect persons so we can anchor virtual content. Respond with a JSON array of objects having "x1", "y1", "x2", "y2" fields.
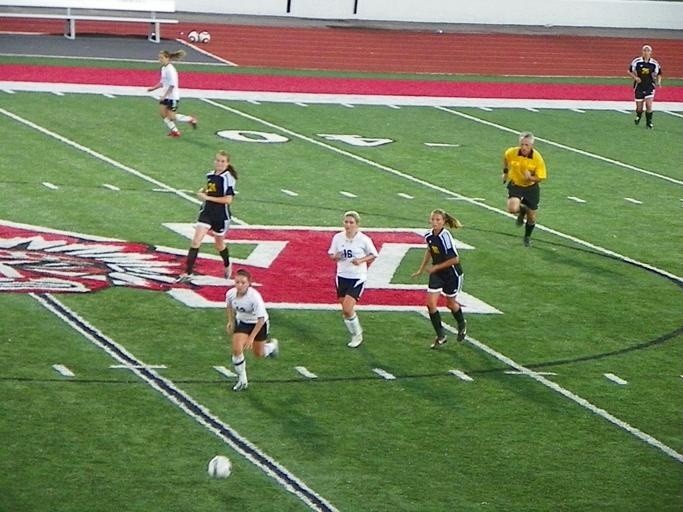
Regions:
[
  {"x1": 327, "y1": 211, "x2": 378, "y2": 348},
  {"x1": 501, "y1": 131, "x2": 546, "y2": 249},
  {"x1": 146, "y1": 49, "x2": 200, "y2": 138},
  {"x1": 410, "y1": 209, "x2": 468, "y2": 349},
  {"x1": 225, "y1": 269, "x2": 279, "y2": 390},
  {"x1": 174, "y1": 149, "x2": 238, "y2": 280},
  {"x1": 627, "y1": 45, "x2": 662, "y2": 128}
]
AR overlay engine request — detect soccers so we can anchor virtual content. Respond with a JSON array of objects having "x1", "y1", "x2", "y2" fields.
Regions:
[
  {"x1": 199, "y1": 30, "x2": 211, "y2": 43},
  {"x1": 207, "y1": 454, "x2": 232, "y2": 480}
]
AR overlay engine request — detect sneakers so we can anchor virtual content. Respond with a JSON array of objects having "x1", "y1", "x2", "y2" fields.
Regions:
[
  {"x1": 456, "y1": 319, "x2": 467, "y2": 342},
  {"x1": 224, "y1": 263, "x2": 231, "y2": 280},
  {"x1": 166, "y1": 131, "x2": 181, "y2": 136},
  {"x1": 173, "y1": 273, "x2": 194, "y2": 283},
  {"x1": 646, "y1": 122, "x2": 654, "y2": 128},
  {"x1": 524, "y1": 237, "x2": 533, "y2": 247},
  {"x1": 192, "y1": 116, "x2": 197, "y2": 129},
  {"x1": 430, "y1": 334, "x2": 448, "y2": 349},
  {"x1": 517, "y1": 207, "x2": 526, "y2": 226},
  {"x1": 232, "y1": 381, "x2": 249, "y2": 392},
  {"x1": 635, "y1": 115, "x2": 641, "y2": 125},
  {"x1": 347, "y1": 334, "x2": 363, "y2": 348},
  {"x1": 269, "y1": 338, "x2": 279, "y2": 360}
]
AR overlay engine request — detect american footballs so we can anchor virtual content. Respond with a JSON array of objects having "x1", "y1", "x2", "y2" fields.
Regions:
[{"x1": 187, "y1": 30, "x2": 199, "y2": 43}]
